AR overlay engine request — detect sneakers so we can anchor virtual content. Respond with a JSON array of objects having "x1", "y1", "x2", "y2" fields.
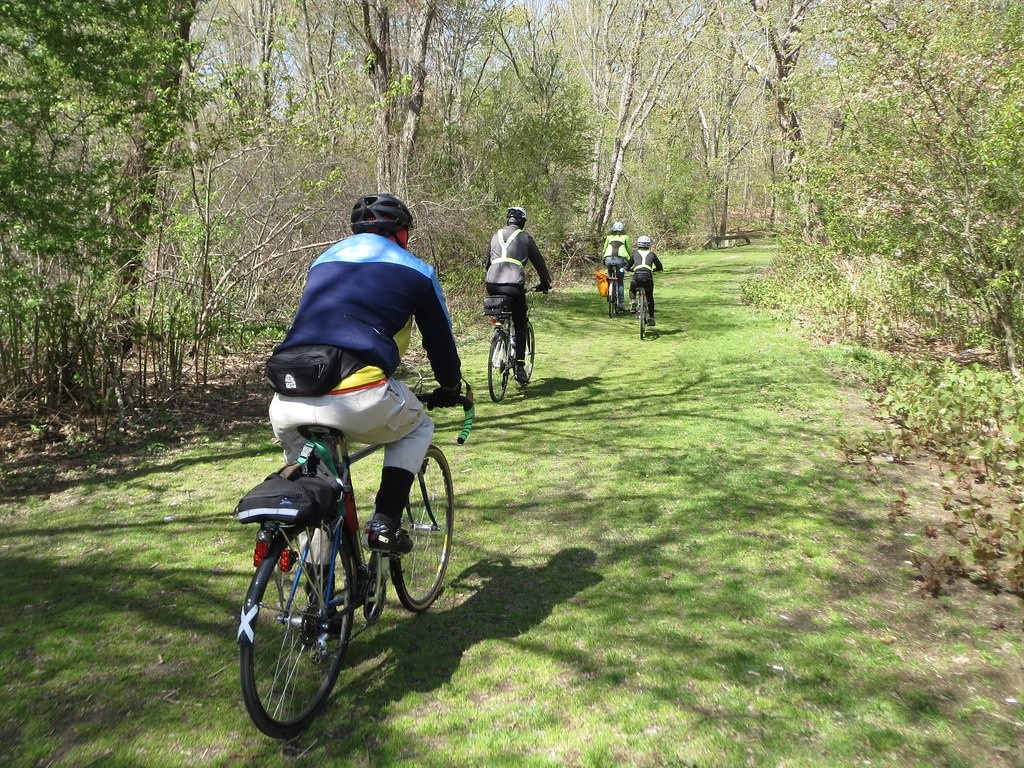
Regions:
[
  {"x1": 328, "y1": 611, "x2": 343, "y2": 640},
  {"x1": 649, "y1": 317, "x2": 655, "y2": 325},
  {"x1": 631, "y1": 299, "x2": 637, "y2": 312},
  {"x1": 516, "y1": 364, "x2": 530, "y2": 383},
  {"x1": 362, "y1": 513, "x2": 414, "y2": 555},
  {"x1": 617, "y1": 304, "x2": 623, "y2": 311}
]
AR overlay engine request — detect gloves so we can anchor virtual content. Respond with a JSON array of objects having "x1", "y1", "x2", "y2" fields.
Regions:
[
  {"x1": 427, "y1": 383, "x2": 461, "y2": 411},
  {"x1": 653, "y1": 268, "x2": 660, "y2": 272},
  {"x1": 541, "y1": 278, "x2": 552, "y2": 293}
]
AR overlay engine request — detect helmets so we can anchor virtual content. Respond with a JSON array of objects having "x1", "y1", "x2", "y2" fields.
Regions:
[
  {"x1": 613, "y1": 223, "x2": 624, "y2": 232},
  {"x1": 638, "y1": 236, "x2": 650, "y2": 247},
  {"x1": 506, "y1": 205, "x2": 527, "y2": 223},
  {"x1": 351, "y1": 193, "x2": 413, "y2": 235}
]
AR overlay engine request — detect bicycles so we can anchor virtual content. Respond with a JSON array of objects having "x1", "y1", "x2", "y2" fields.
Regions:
[
  {"x1": 233, "y1": 390, "x2": 476, "y2": 741},
  {"x1": 605, "y1": 262, "x2": 619, "y2": 318},
  {"x1": 484, "y1": 279, "x2": 553, "y2": 403},
  {"x1": 625, "y1": 265, "x2": 663, "y2": 342}
]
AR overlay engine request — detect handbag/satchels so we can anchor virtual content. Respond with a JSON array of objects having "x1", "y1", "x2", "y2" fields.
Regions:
[
  {"x1": 633, "y1": 272, "x2": 651, "y2": 281},
  {"x1": 484, "y1": 294, "x2": 508, "y2": 316},
  {"x1": 595, "y1": 267, "x2": 608, "y2": 298},
  {"x1": 265, "y1": 341, "x2": 392, "y2": 398},
  {"x1": 235, "y1": 461, "x2": 343, "y2": 526},
  {"x1": 605, "y1": 257, "x2": 625, "y2": 266}
]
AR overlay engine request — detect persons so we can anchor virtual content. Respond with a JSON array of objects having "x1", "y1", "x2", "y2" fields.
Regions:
[
  {"x1": 485, "y1": 206, "x2": 551, "y2": 384},
  {"x1": 264, "y1": 194, "x2": 462, "y2": 635},
  {"x1": 601, "y1": 220, "x2": 634, "y2": 303},
  {"x1": 624, "y1": 235, "x2": 663, "y2": 326}
]
